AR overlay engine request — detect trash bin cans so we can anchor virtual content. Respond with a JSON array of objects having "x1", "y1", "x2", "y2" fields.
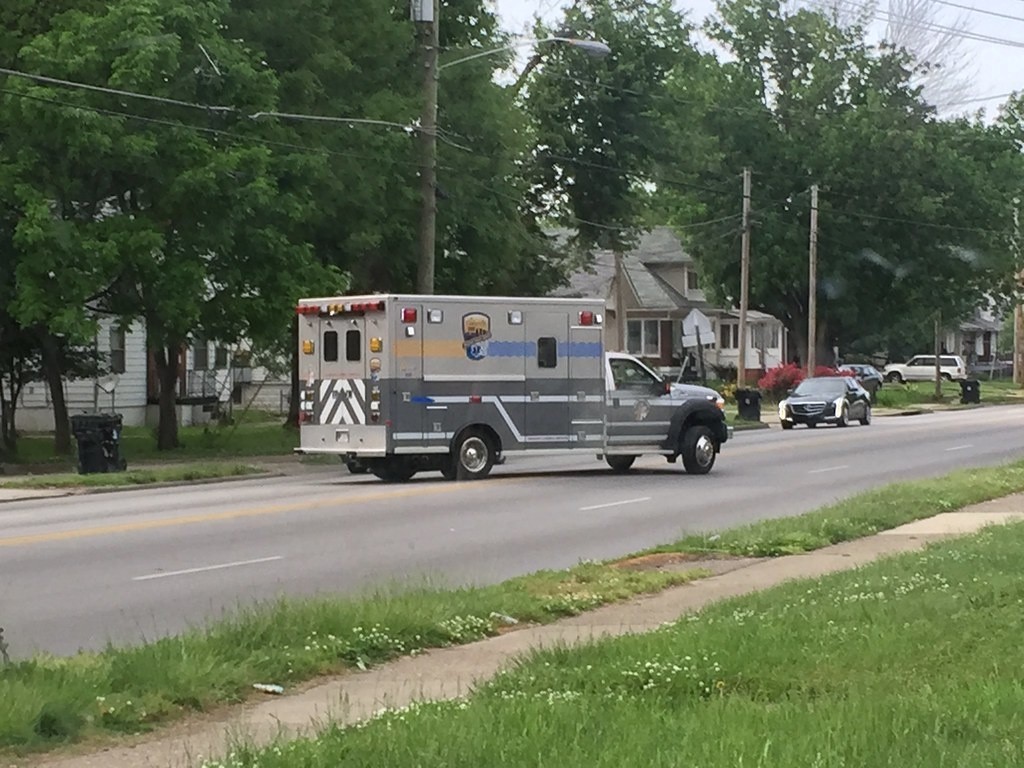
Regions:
[
  {"x1": 70, "y1": 413, "x2": 127, "y2": 476},
  {"x1": 959, "y1": 379, "x2": 981, "y2": 405},
  {"x1": 732, "y1": 387, "x2": 763, "y2": 422}
]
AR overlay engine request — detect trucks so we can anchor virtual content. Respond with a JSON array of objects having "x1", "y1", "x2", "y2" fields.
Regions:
[{"x1": 293, "y1": 293, "x2": 734, "y2": 480}]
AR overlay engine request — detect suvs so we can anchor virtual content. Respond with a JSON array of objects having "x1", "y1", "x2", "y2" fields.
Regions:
[{"x1": 883, "y1": 354, "x2": 969, "y2": 384}]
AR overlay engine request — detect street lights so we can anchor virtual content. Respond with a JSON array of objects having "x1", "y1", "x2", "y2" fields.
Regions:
[{"x1": 418, "y1": 36, "x2": 612, "y2": 294}]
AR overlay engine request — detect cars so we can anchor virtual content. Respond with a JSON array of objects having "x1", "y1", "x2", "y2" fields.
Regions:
[
  {"x1": 837, "y1": 364, "x2": 883, "y2": 392},
  {"x1": 778, "y1": 375, "x2": 871, "y2": 429}
]
